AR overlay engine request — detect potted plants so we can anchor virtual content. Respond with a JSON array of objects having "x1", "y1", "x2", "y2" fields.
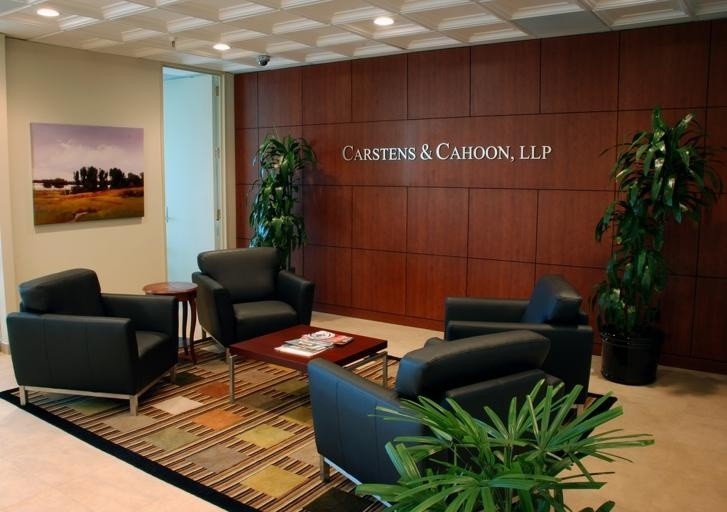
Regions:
[{"x1": 583, "y1": 101, "x2": 724, "y2": 388}]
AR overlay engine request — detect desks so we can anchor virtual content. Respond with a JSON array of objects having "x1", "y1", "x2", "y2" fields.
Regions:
[{"x1": 139, "y1": 279, "x2": 201, "y2": 364}]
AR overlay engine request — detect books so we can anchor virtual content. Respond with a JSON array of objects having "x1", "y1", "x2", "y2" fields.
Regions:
[{"x1": 273, "y1": 328, "x2": 353, "y2": 358}]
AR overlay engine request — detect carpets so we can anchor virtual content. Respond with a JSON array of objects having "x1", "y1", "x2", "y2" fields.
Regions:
[{"x1": 0, "y1": 333, "x2": 618, "y2": 512}]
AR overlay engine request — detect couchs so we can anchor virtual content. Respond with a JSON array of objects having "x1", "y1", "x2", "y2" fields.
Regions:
[
  {"x1": 187, "y1": 246, "x2": 314, "y2": 360},
  {"x1": 301, "y1": 328, "x2": 567, "y2": 512},
  {"x1": 5, "y1": 265, "x2": 180, "y2": 418},
  {"x1": 443, "y1": 268, "x2": 593, "y2": 410}
]
